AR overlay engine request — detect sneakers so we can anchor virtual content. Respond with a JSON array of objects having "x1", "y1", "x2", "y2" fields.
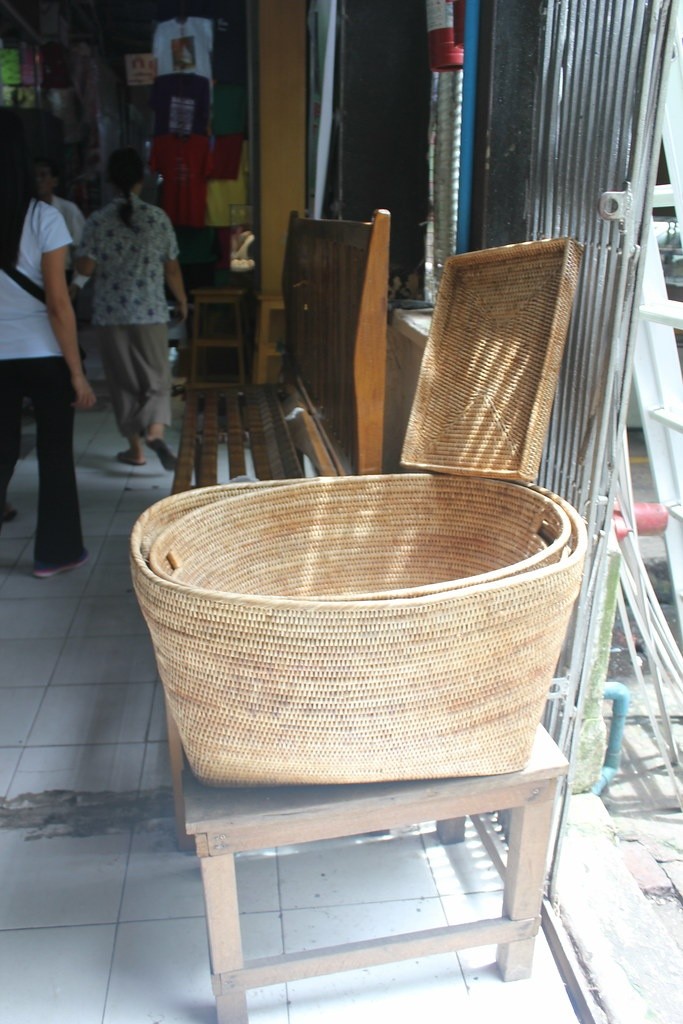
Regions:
[
  {"x1": 2, "y1": 503, "x2": 17, "y2": 522},
  {"x1": 33, "y1": 549, "x2": 90, "y2": 578}
]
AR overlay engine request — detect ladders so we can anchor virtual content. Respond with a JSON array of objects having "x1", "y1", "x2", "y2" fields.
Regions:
[{"x1": 629, "y1": 49, "x2": 683, "y2": 630}]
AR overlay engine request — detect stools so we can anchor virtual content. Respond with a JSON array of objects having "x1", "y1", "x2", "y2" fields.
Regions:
[{"x1": 188, "y1": 277, "x2": 248, "y2": 382}]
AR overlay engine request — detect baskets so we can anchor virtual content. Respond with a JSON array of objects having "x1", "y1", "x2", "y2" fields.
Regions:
[{"x1": 129, "y1": 472, "x2": 587, "y2": 789}]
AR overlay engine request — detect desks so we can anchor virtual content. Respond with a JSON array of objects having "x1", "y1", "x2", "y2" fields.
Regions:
[{"x1": 181, "y1": 722, "x2": 569, "y2": 1024}]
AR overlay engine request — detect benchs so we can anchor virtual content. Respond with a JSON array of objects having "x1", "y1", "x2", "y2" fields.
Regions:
[{"x1": 170, "y1": 209, "x2": 391, "y2": 493}]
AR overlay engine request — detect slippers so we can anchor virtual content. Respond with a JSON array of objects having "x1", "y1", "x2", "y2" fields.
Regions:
[
  {"x1": 151, "y1": 438, "x2": 177, "y2": 469},
  {"x1": 117, "y1": 449, "x2": 146, "y2": 466}
]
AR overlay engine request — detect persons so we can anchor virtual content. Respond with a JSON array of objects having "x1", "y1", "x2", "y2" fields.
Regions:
[
  {"x1": 68, "y1": 147, "x2": 188, "y2": 466},
  {"x1": 0, "y1": 106, "x2": 91, "y2": 579}
]
